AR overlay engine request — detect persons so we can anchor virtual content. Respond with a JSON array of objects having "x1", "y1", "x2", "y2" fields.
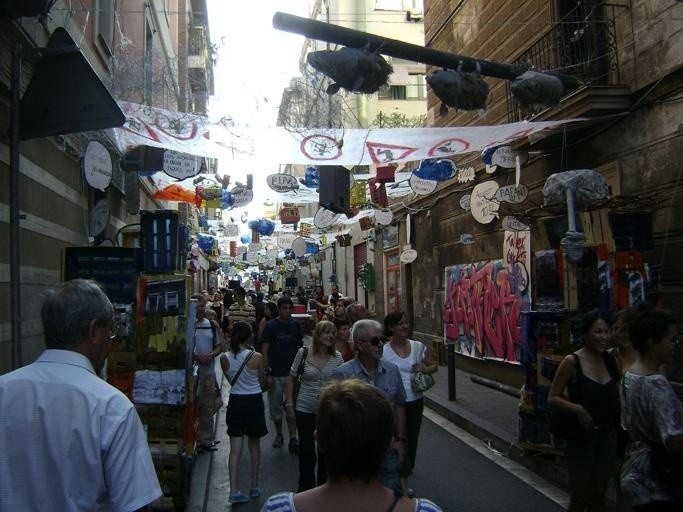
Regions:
[
  {"x1": 179, "y1": 275, "x2": 443, "y2": 512},
  {"x1": 0, "y1": 279, "x2": 163, "y2": 512},
  {"x1": 548, "y1": 306, "x2": 682, "y2": 512}
]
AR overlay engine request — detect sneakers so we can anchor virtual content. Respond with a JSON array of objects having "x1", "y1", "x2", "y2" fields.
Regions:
[
  {"x1": 229, "y1": 492, "x2": 250, "y2": 504},
  {"x1": 272, "y1": 435, "x2": 284, "y2": 447},
  {"x1": 250, "y1": 487, "x2": 260, "y2": 497},
  {"x1": 289, "y1": 438, "x2": 299, "y2": 452}
]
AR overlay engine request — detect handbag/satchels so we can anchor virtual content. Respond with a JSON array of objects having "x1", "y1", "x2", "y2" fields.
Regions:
[
  {"x1": 194, "y1": 373, "x2": 223, "y2": 416},
  {"x1": 284, "y1": 380, "x2": 301, "y2": 411},
  {"x1": 412, "y1": 370, "x2": 436, "y2": 393}
]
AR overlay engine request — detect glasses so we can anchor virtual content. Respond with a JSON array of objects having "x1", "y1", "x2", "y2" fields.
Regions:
[
  {"x1": 394, "y1": 321, "x2": 410, "y2": 327},
  {"x1": 362, "y1": 335, "x2": 386, "y2": 346},
  {"x1": 95, "y1": 320, "x2": 121, "y2": 340}
]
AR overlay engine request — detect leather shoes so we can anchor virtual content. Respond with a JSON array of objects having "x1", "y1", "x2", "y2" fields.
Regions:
[{"x1": 201, "y1": 440, "x2": 221, "y2": 451}]
